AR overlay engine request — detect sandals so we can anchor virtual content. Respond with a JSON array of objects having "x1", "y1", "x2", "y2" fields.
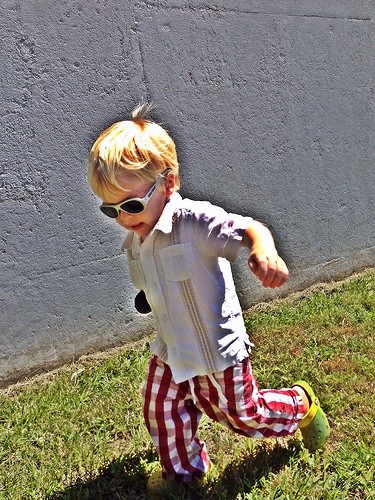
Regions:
[
  {"x1": 291, "y1": 380, "x2": 330, "y2": 452},
  {"x1": 146, "y1": 460, "x2": 216, "y2": 497}
]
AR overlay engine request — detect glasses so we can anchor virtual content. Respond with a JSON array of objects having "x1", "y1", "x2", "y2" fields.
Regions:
[{"x1": 99, "y1": 169, "x2": 170, "y2": 219}]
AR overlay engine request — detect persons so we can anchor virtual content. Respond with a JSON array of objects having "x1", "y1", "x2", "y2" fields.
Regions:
[{"x1": 88, "y1": 95, "x2": 331, "y2": 499}]
